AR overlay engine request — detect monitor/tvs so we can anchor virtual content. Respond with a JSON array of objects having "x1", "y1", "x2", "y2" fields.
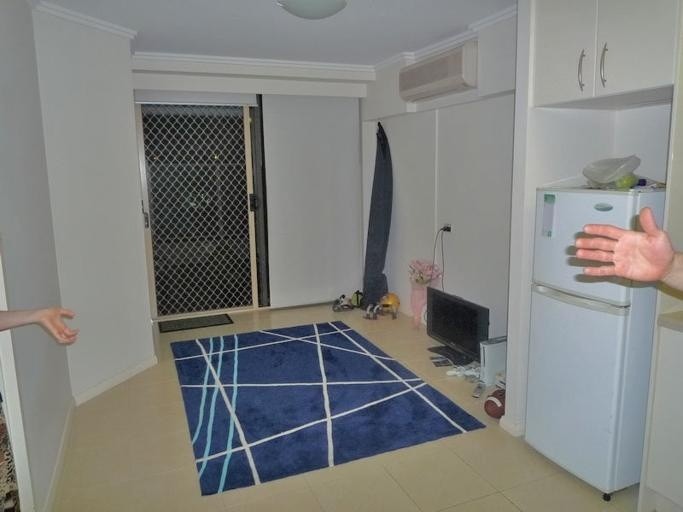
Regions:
[{"x1": 426, "y1": 287, "x2": 489, "y2": 366}]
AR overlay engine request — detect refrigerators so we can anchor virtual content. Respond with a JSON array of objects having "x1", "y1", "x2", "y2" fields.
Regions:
[{"x1": 524, "y1": 182, "x2": 664, "y2": 501}]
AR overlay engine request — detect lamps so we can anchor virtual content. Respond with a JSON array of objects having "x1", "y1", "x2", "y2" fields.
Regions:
[{"x1": 277, "y1": 0, "x2": 348, "y2": 20}]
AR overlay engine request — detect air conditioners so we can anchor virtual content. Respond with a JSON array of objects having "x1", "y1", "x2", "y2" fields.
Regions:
[{"x1": 398, "y1": 38, "x2": 478, "y2": 100}]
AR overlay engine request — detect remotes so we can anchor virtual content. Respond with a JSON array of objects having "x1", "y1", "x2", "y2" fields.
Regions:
[{"x1": 473, "y1": 384, "x2": 483, "y2": 397}]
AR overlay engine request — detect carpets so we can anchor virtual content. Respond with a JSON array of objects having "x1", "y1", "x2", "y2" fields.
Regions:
[
  {"x1": 171, "y1": 320, "x2": 485, "y2": 495},
  {"x1": 158, "y1": 313, "x2": 233, "y2": 333}
]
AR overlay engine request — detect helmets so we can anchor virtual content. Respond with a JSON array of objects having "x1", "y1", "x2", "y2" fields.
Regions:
[{"x1": 380, "y1": 293, "x2": 400, "y2": 311}]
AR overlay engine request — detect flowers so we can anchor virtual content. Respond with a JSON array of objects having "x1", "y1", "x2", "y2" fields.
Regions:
[{"x1": 407, "y1": 261, "x2": 442, "y2": 326}]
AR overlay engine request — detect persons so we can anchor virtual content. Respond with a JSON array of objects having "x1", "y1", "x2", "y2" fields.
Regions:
[
  {"x1": 574, "y1": 207, "x2": 683, "y2": 294},
  {"x1": 0, "y1": 306, "x2": 81, "y2": 346}
]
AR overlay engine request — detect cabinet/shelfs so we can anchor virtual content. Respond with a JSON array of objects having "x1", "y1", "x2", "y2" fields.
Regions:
[
  {"x1": 634, "y1": 70, "x2": 682, "y2": 512},
  {"x1": 533, "y1": 0, "x2": 682, "y2": 109}
]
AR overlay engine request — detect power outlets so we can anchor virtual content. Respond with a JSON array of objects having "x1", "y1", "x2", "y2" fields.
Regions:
[{"x1": 441, "y1": 222, "x2": 455, "y2": 238}]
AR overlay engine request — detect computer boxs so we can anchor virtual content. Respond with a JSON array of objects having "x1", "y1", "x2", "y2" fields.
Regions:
[{"x1": 479, "y1": 337, "x2": 506, "y2": 386}]
